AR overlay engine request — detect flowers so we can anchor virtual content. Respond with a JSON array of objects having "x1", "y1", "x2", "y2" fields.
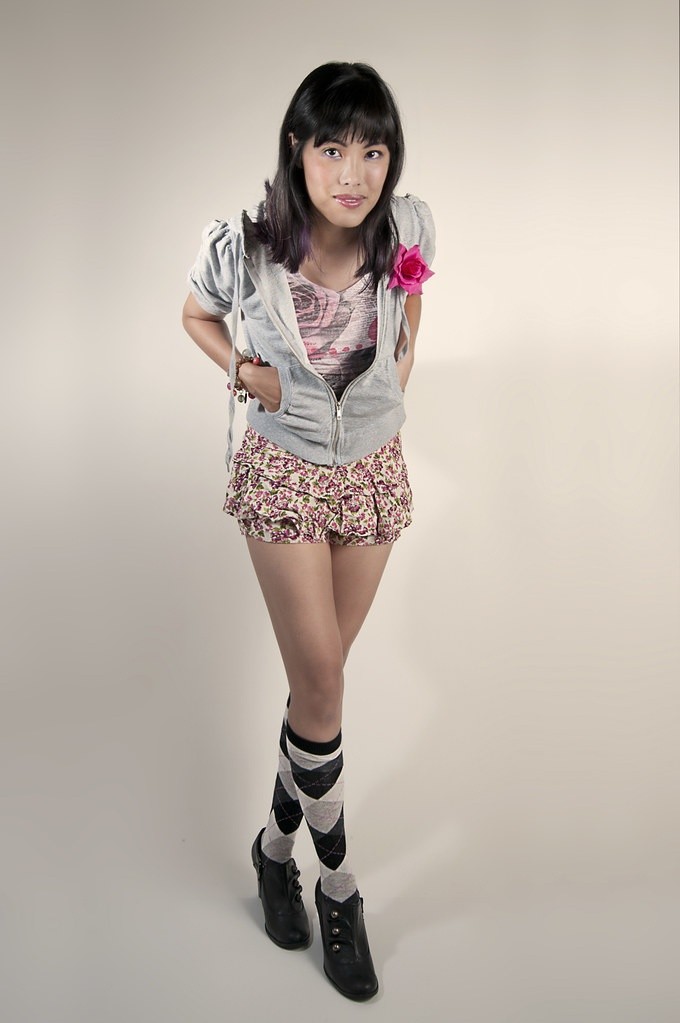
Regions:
[{"x1": 386, "y1": 241, "x2": 435, "y2": 298}]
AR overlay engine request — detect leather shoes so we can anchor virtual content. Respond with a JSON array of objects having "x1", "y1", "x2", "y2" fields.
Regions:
[
  {"x1": 251, "y1": 827, "x2": 311, "y2": 950},
  {"x1": 314, "y1": 876, "x2": 379, "y2": 998}
]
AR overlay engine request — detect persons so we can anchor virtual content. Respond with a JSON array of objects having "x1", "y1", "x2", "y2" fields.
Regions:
[{"x1": 184, "y1": 62, "x2": 438, "y2": 1001}]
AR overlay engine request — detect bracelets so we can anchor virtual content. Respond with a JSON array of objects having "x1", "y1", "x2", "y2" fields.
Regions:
[{"x1": 226, "y1": 354, "x2": 263, "y2": 403}]
